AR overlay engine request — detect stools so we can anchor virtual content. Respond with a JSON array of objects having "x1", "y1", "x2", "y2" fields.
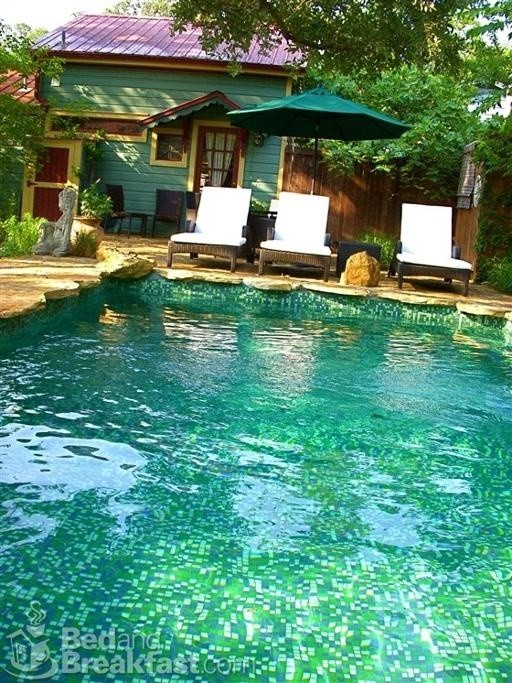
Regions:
[{"x1": 336, "y1": 241, "x2": 382, "y2": 277}]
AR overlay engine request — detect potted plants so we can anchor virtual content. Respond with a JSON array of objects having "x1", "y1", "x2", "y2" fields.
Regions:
[{"x1": 74, "y1": 178, "x2": 114, "y2": 227}]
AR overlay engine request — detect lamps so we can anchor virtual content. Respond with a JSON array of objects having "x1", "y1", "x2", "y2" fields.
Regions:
[{"x1": 253, "y1": 132, "x2": 269, "y2": 146}]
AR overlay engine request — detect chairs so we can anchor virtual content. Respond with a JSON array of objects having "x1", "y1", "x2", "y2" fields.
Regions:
[
  {"x1": 151, "y1": 189, "x2": 183, "y2": 238},
  {"x1": 389, "y1": 203, "x2": 474, "y2": 296},
  {"x1": 259, "y1": 192, "x2": 331, "y2": 282},
  {"x1": 104, "y1": 184, "x2": 147, "y2": 238},
  {"x1": 167, "y1": 187, "x2": 254, "y2": 272}
]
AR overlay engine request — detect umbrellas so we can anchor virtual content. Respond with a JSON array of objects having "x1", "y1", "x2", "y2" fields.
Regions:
[{"x1": 224, "y1": 86, "x2": 411, "y2": 194}]
